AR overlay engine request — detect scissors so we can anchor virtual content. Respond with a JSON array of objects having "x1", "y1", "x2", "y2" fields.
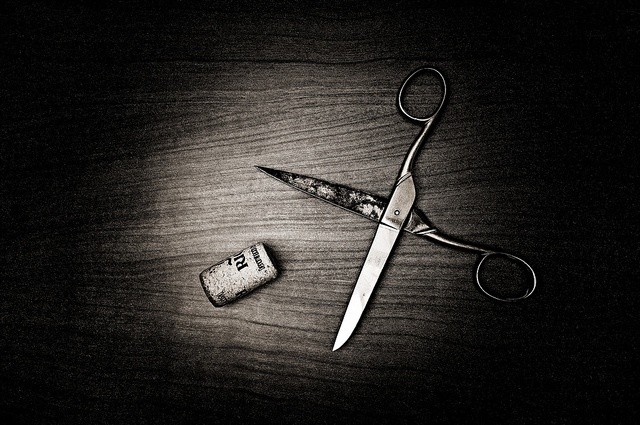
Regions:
[{"x1": 254, "y1": 65, "x2": 537, "y2": 352}]
